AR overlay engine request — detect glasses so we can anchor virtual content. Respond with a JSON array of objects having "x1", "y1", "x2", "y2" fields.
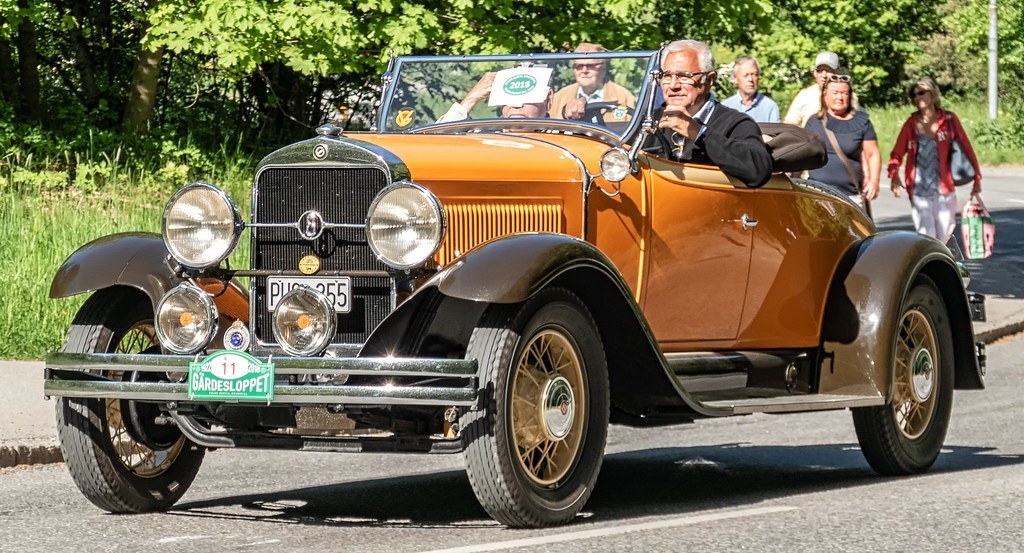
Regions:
[
  {"x1": 576, "y1": 63, "x2": 605, "y2": 70},
  {"x1": 655, "y1": 69, "x2": 709, "y2": 85},
  {"x1": 912, "y1": 90, "x2": 930, "y2": 99},
  {"x1": 825, "y1": 74, "x2": 852, "y2": 88}
]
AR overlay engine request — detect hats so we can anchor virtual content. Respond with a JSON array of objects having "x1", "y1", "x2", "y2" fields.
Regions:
[
  {"x1": 815, "y1": 51, "x2": 840, "y2": 70},
  {"x1": 573, "y1": 42, "x2": 605, "y2": 67}
]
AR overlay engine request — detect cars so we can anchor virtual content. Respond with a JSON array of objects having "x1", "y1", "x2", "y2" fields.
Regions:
[{"x1": 45, "y1": 51, "x2": 987, "y2": 529}]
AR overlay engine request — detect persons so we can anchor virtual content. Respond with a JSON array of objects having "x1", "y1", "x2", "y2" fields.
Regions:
[
  {"x1": 783, "y1": 52, "x2": 859, "y2": 178},
  {"x1": 437, "y1": 63, "x2": 572, "y2": 137},
  {"x1": 719, "y1": 56, "x2": 780, "y2": 124},
  {"x1": 887, "y1": 78, "x2": 981, "y2": 244},
  {"x1": 565, "y1": 40, "x2": 772, "y2": 188},
  {"x1": 807, "y1": 74, "x2": 881, "y2": 209},
  {"x1": 551, "y1": 44, "x2": 635, "y2": 124}
]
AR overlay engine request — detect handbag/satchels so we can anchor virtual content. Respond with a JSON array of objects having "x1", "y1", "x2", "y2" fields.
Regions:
[
  {"x1": 860, "y1": 192, "x2": 871, "y2": 221},
  {"x1": 946, "y1": 110, "x2": 975, "y2": 186},
  {"x1": 961, "y1": 190, "x2": 995, "y2": 260}
]
